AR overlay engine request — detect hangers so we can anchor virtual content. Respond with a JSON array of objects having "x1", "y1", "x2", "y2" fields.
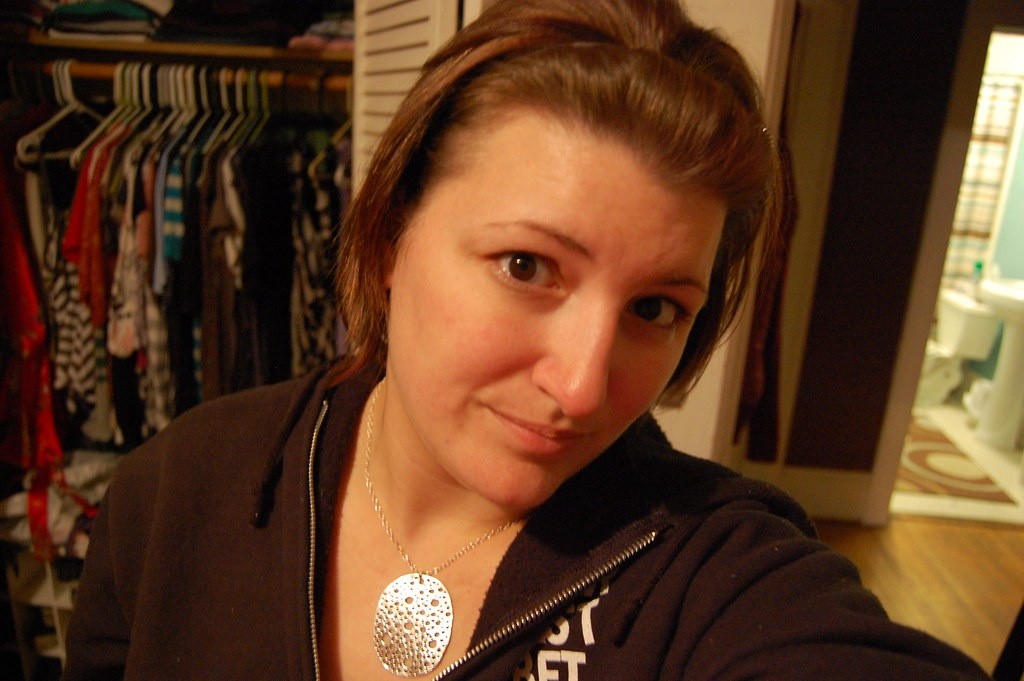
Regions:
[{"x1": 11, "y1": 60, "x2": 357, "y2": 176}]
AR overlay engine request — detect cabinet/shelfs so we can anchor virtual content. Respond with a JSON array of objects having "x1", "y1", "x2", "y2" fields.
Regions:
[
  {"x1": 6, "y1": 520, "x2": 91, "y2": 678},
  {"x1": 351, "y1": 0, "x2": 792, "y2": 479}
]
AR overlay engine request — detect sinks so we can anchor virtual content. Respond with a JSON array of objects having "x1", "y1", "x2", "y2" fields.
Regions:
[{"x1": 977, "y1": 280, "x2": 1024, "y2": 315}]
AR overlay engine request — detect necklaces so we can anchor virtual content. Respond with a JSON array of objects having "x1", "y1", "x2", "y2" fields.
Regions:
[{"x1": 361, "y1": 394, "x2": 515, "y2": 676}]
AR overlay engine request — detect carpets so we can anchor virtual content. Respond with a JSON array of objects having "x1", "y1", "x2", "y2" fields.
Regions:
[{"x1": 891, "y1": 412, "x2": 1016, "y2": 504}]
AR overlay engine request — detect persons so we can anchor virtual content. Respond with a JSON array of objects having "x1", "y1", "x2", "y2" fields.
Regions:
[{"x1": 59, "y1": 0, "x2": 989, "y2": 681}]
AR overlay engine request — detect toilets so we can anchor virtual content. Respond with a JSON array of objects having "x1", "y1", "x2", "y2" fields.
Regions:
[{"x1": 913, "y1": 288, "x2": 1001, "y2": 406}]
